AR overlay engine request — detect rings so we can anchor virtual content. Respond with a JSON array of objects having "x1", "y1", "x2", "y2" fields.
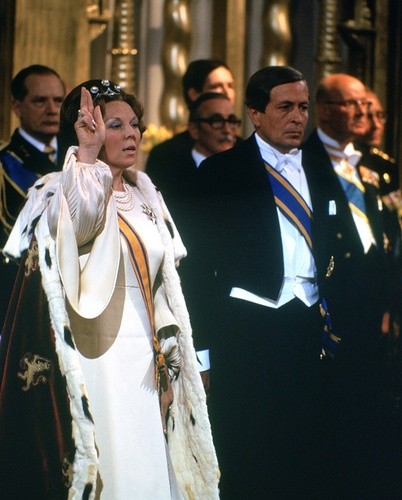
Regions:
[{"x1": 78, "y1": 110, "x2": 81, "y2": 117}]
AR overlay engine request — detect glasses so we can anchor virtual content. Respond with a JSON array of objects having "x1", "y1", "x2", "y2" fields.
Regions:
[
  {"x1": 368, "y1": 111, "x2": 387, "y2": 124},
  {"x1": 327, "y1": 100, "x2": 372, "y2": 110},
  {"x1": 199, "y1": 114, "x2": 242, "y2": 130}
]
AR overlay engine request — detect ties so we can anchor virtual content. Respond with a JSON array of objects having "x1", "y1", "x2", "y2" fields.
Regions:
[{"x1": 44, "y1": 145, "x2": 57, "y2": 165}]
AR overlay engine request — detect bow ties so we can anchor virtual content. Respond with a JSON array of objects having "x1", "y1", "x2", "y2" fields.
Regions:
[
  {"x1": 346, "y1": 151, "x2": 362, "y2": 167},
  {"x1": 272, "y1": 148, "x2": 302, "y2": 173}
]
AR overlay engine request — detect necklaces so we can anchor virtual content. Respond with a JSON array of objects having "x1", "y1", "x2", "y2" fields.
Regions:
[{"x1": 108, "y1": 175, "x2": 135, "y2": 211}]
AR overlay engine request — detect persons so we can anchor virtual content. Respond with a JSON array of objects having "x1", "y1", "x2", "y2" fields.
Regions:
[
  {"x1": 34, "y1": 79, "x2": 221, "y2": 500},
  {"x1": 144, "y1": 59, "x2": 247, "y2": 191},
  {"x1": 173, "y1": 92, "x2": 246, "y2": 184},
  {"x1": 189, "y1": 66, "x2": 329, "y2": 500},
  {"x1": 297, "y1": 72, "x2": 402, "y2": 500},
  {"x1": 0, "y1": 64, "x2": 67, "y2": 500}
]
